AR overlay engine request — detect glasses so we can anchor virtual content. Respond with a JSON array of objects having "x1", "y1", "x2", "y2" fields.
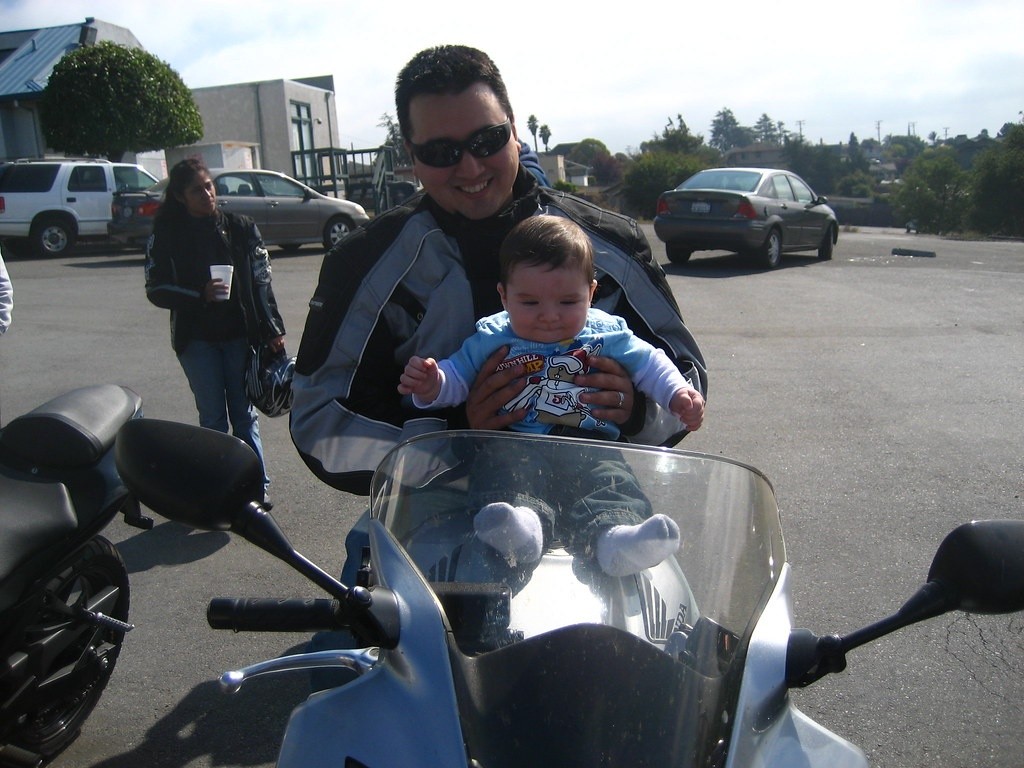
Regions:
[{"x1": 411, "y1": 117, "x2": 512, "y2": 167}]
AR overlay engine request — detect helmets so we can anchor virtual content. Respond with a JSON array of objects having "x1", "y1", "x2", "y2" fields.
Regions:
[{"x1": 244, "y1": 343, "x2": 298, "y2": 418}]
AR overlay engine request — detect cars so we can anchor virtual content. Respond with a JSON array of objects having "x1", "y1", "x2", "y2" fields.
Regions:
[
  {"x1": 906, "y1": 219, "x2": 936, "y2": 234},
  {"x1": 654, "y1": 168, "x2": 839, "y2": 269},
  {"x1": 107, "y1": 169, "x2": 370, "y2": 252}
]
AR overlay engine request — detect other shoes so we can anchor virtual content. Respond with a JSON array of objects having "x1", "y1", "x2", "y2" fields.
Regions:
[{"x1": 264, "y1": 493, "x2": 272, "y2": 509}]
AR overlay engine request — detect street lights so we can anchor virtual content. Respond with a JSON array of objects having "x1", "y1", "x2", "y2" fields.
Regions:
[{"x1": 324, "y1": 91, "x2": 338, "y2": 198}]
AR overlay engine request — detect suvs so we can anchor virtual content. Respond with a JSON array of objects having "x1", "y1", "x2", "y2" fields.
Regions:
[{"x1": 0, "y1": 157, "x2": 161, "y2": 258}]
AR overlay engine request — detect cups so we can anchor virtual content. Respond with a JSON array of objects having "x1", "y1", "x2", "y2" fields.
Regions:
[{"x1": 210, "y1": 265, "x2": 234, "y2": 300}]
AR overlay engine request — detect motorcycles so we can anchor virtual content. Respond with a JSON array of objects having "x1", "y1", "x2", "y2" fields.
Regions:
[
  {"x1": 113, "y1": 414, "x2": 1024, "y2": 768},
  {"x1": 0, "y1": 384, "x2": 154, "y2": 768}
]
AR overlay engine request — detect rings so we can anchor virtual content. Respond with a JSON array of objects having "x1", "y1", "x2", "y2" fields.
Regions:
[{"x1": 618, "y1": 392, "x2": 624, "y2": 406}]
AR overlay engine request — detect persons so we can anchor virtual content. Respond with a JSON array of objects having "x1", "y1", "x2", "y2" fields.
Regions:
[
  {"x1": 288, "y1": 44, "x2": 708, "y2": 695},
  {"x1": 0, "y1": 254, "x2": 14, "y2": 335},
  {"x1": 144, "y1": 159, "x2": 285, "y2": 510},
  {"x1": 398, "y1": 215, "x2": 705, "y2": 575}
]
